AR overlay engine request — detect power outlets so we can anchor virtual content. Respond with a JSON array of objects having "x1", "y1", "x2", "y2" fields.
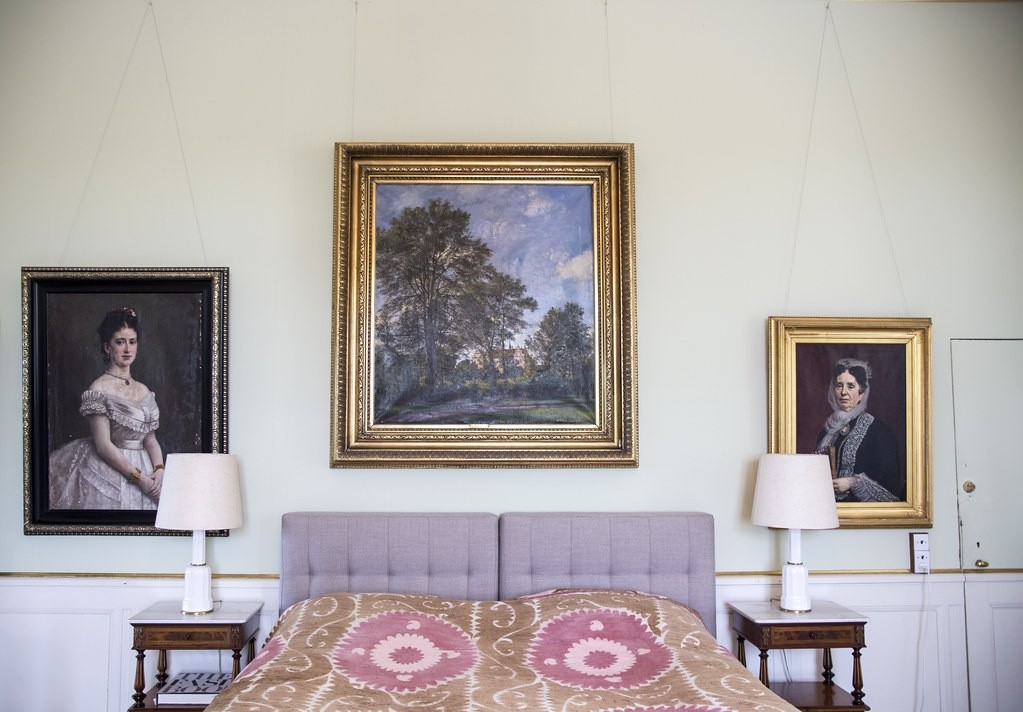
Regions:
[{"x1": 909, "y1": 533, "x2": 930, "y2": 573}]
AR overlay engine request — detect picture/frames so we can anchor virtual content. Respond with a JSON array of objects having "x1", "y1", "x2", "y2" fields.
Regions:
[
  {"x1": 21, "y1": 265, "x2": 229, "y2": 537},
  {"x1": 330, "y1": 144, "x2": 640, "y2": 468},
  {"x1": 765, "y1": 314, "x2": 934, "y2": 529}
]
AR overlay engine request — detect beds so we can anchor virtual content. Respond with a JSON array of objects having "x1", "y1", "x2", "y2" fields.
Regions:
[{"x1": 201, "y1": 511, "x2": 803, "y2": 712}]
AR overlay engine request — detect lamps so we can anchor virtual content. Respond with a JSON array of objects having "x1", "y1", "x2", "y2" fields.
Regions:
[
  {"x1": 750, "y1": 454, "x2": 838, "y2": 612},
  {"x1": 155, "y1": 452, "x2": 241, "y2": 615}
]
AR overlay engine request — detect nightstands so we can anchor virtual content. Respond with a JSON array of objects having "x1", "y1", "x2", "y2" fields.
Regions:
[
  {"x1": 728, "y1": 597, "x2": 868, "y2": 712},
  {"x1": 127, "y1": 600, "x2": 264, "y2": 712}
]
